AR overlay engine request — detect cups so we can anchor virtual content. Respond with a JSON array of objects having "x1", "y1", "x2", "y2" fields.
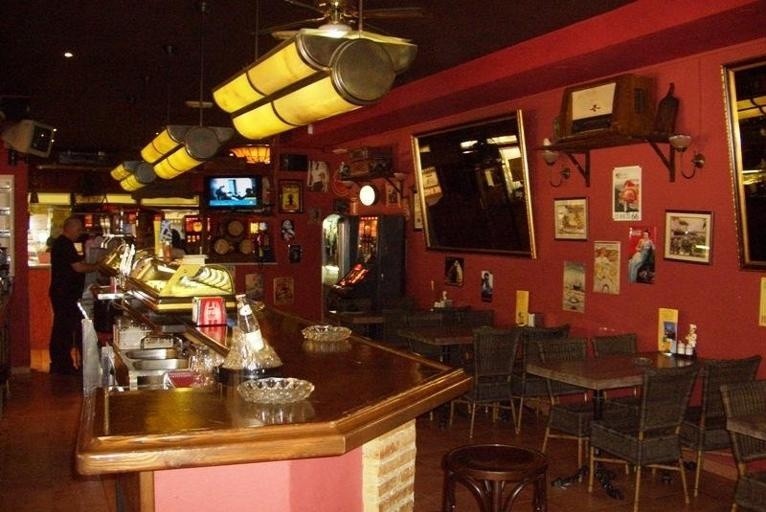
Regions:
[
  {"x1": 188, "y1": 353, "x2": 221, "y2": 387},
  {"x1": 161, "y1": 239, "x2": 173, "y2": 257}
]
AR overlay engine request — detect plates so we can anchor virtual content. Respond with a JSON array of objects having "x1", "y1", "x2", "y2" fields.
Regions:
[
  {"x1": 298, "y1": 324, "x2": 353, "y2": 344},
  {"x1": 235, "y1": 376, "x2": 313, "y2": 403},
  {"x1": 213, "y1": 220, "x2": 252, "y2": 257}
]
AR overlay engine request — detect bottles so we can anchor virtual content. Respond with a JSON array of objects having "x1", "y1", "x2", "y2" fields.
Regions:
[
  {"x1": 676, "y1": 339, "x2": 693, "y2": 356},
  {"x1": 361, "y1": 233, "x2": 372, "y2": 255},
  {"x1": 234, "y1": 292, "x2": 265, "y2": 354}
]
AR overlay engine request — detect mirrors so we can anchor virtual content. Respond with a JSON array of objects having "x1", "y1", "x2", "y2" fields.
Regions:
[
  {"x1": 409, "y1": 109, "x2": 537, "y2": 261},
  {"x1": 718, "y1": 54, "x2": 765, "y2": 272}
]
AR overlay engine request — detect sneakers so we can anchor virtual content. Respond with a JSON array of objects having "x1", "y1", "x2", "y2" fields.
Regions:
[{"x1": 49, "y1": 364, "x2": 78, "y2": 377}]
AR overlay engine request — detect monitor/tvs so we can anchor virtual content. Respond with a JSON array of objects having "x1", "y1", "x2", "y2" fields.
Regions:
[
  {"x1": 203, "y1": 173, "x2": 263, "y2": 210},
  {"x1": 1, "y1": 119, "x2": 55, "y2": 159}
]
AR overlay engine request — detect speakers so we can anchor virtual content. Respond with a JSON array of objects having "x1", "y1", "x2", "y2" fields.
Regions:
[{"x1": 558, "y1": 73, "x2": 657, "y2": 140}]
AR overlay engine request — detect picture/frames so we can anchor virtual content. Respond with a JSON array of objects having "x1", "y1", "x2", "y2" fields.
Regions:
[
  {"x1": 664, "y1": 209, "x2": 713, "y2": 266},
  {"x1": 278, "y1": 178, "x2": 304, "y2": 214},
  {"x1": 553, "y1": 196, "x2": 590, "y2": 242}
]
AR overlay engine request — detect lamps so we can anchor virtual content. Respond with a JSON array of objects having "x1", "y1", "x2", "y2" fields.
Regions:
[
  {"x1": 140, "y1": 1, "x2": 234, "y2": 180},
  {"x1": 211, "y1": 0, "x2": 420, "y2": 140},
  {"x1": 539, "y1": 138, "x2": 570, "y2": 189},
  {"x1": 669, "y1": 135, "x2": 704, "y2": 180}
]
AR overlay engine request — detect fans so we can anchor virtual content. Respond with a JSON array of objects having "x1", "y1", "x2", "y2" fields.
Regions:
[{"x1": 250, "y1": 0, "x2": 426, "y2": 46}]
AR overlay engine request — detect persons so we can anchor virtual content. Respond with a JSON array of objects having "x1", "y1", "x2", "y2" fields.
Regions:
[
  {"x1": 45, "y1": 214, "x2": 108, "y2": 379},
  {"x1": 445, "y1": 259, "x2": 464, "y2": 286},
  {"x1": 684, "y1": 324, "x2": 699, "y2": 349},
  {"x1": 480, "y1": 272, "x2": 493, "y2": 299},
  {"x1": 234, "y1": 189, "x2": 254, "y2": 201},
  {"x1": 215, "y1": 184, "x2": 226, "y2": 199},
  {"x1": 593, "y1": 247, "x2": 612, "y2": 286},
  {"x1": 626, "y1": 230, "x2": 655, "y2": 282},
  {"x1": 623, "y1": 179, "x2": 637, "y2": 211},
  {"x1": 308, "y1": 161, "x2": 326, "y2": 193}
]
